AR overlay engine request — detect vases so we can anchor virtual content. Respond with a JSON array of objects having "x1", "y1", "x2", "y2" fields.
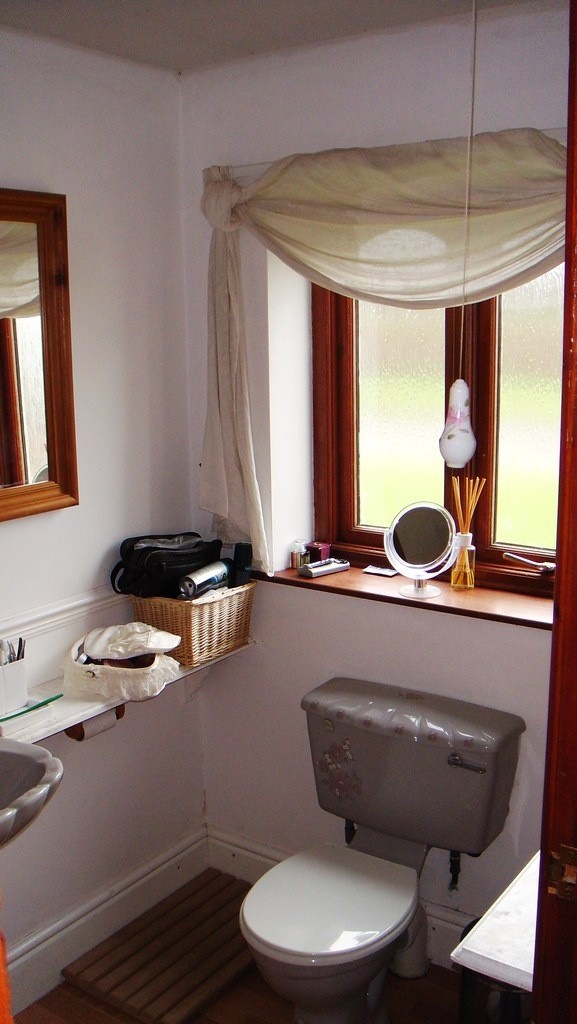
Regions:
[{"x1": 451, "y1": 532, "x2": 475, "y2": 588}]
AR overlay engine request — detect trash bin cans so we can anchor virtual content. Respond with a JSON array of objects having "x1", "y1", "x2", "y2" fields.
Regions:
[{"x1": 458, "y1": 915, "x2": 533, "y2": 1024}]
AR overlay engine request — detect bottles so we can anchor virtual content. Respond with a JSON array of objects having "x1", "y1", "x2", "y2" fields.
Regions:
[{"x1": 183, "y1": 558, "x2": 232, "y2": 600}]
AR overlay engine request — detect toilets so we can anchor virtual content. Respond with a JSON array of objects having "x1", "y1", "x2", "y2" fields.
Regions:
[{"x1": 237, "y1": 677, "x2": 527, "y2": 1024}]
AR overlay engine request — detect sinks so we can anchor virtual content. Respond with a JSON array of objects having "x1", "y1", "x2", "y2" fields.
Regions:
[{"x1": 0, "y1": 737, "x2": 64, "y2": 847}]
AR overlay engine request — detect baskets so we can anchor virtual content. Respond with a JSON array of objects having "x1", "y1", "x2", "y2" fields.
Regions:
[{"x1": 129, "y1": 579, "x2": 257, "y2": 669}]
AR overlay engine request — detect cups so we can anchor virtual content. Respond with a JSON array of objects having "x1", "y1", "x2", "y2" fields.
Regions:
[{"x1": 0, "y1": 656, "x2": 29, "y2": 717}]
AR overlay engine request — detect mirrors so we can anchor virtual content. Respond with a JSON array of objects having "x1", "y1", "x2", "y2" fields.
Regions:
[{"x1": 0, "y1": 188, "x2": 80, "y2": 523}]
